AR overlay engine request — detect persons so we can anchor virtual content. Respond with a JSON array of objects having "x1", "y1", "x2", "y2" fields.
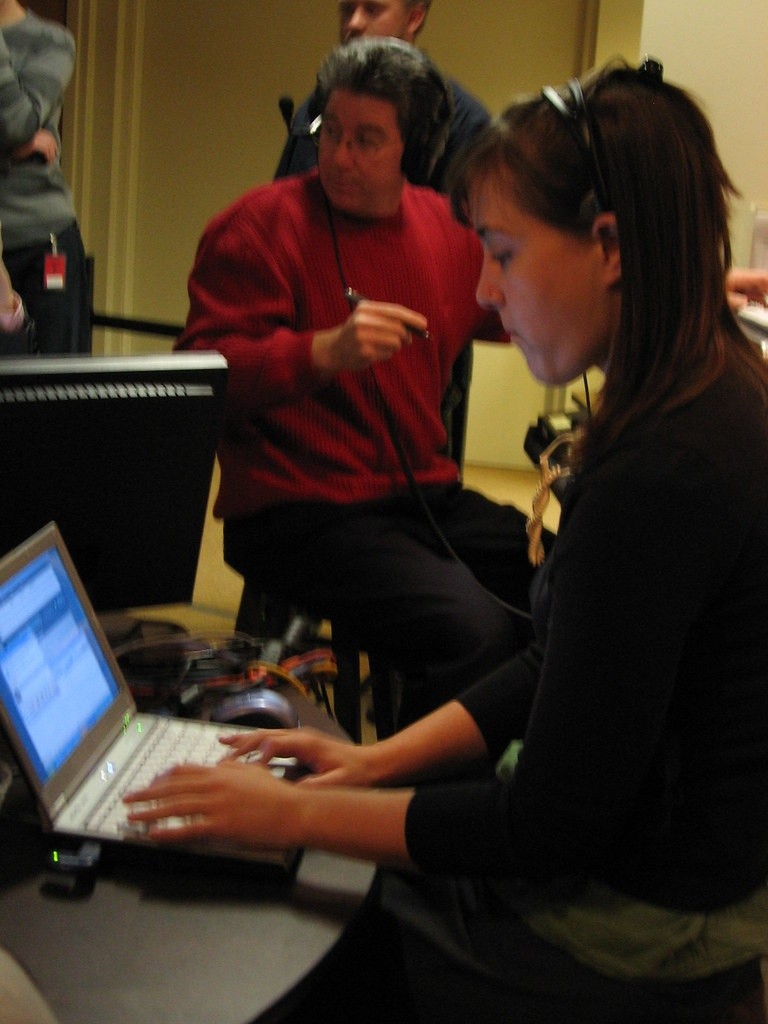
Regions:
[
  {"x1": 173, "y1": 0, "x2": 768, "y2": 733},
  {"x1": 122, "y1": 54, "x2": 768, "y2": 1024},
  {"x1": 0, "y1": 0, "x2": 85, "y2": 357}
]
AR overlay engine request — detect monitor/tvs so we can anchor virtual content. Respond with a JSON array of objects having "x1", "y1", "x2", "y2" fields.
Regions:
[{"x1": 0, "y1": 352, "x2": 228, "y2": 613}]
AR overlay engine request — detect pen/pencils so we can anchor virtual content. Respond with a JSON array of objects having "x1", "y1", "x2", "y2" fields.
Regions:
[{"x1": 344, "y1": 288, "x2": 431, "y2": 337}]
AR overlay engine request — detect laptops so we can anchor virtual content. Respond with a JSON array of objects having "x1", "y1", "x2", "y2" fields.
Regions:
[{"x1": 0, "y1": 519, "x2": 308, "y2": 867}]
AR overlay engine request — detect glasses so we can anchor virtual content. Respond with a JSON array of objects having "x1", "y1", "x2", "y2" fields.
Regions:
[{"x1": 309, "y1": 113, "x2": 400, "y2": 158}]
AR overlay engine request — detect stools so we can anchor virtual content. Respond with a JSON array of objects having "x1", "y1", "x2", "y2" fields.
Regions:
[{"x1": 231, "y1": 583, "x2": 403, "y2": 749}]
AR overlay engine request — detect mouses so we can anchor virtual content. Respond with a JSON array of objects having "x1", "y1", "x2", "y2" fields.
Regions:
[{"x1": 211, "y1": 687, "x2": 299, "y2": 731}]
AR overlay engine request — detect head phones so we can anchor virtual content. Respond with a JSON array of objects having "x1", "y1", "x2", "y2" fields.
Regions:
[{"x1": 306, "y1": 41, "x2": 454, "y2": 185}]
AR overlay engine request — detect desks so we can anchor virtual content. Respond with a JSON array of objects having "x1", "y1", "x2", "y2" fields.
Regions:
[{"x1": 4, "y1": 627, "x2": 387, "y2": 1023}]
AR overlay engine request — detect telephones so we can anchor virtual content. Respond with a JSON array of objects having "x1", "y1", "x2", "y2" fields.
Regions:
[{"x1": 524, "y1": 393, "x2": 584, "y2": 481}]
